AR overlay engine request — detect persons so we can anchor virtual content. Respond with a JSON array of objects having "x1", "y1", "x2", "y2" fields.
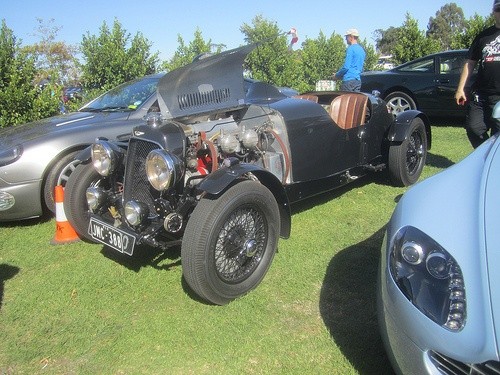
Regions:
[
  {"x1": 332, "y1": 28, "x2": 367, "y2": 92},
  {"x1": 455, "y1": 0, "x2": 500, "y2": 147}
]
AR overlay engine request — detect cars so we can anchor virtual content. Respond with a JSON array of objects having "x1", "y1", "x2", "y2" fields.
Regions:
[
  {"x1": 65, "y1": 38, "x2": 426, "y2": 306},
  {"x1": 358, "y1": 49, "x2": 478, "y2": 124},
  {"x1": 1, "y1": 73, "x2": 300, "y2": 227},
  {"x1": 379, "y1": 132, "x2": 500, "y2": 374}
]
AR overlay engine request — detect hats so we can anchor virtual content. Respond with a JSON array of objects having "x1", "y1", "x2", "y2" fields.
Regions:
[
  {"x1": 343, "y1": 28, "x2": 360, "y2": 36},
  {"x1": 492, "y1": 0, "x2": 500, "y2": 10}
]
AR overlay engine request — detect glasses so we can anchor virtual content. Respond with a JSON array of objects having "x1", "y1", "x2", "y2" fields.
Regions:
[{"x1": 492, "y1": 7, "x2": 500, "y2": 13}]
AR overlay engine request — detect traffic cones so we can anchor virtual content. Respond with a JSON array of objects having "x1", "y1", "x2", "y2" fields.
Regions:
[{"x1": 50, "y1": 185, "x2": 80, "y2": 246}]
